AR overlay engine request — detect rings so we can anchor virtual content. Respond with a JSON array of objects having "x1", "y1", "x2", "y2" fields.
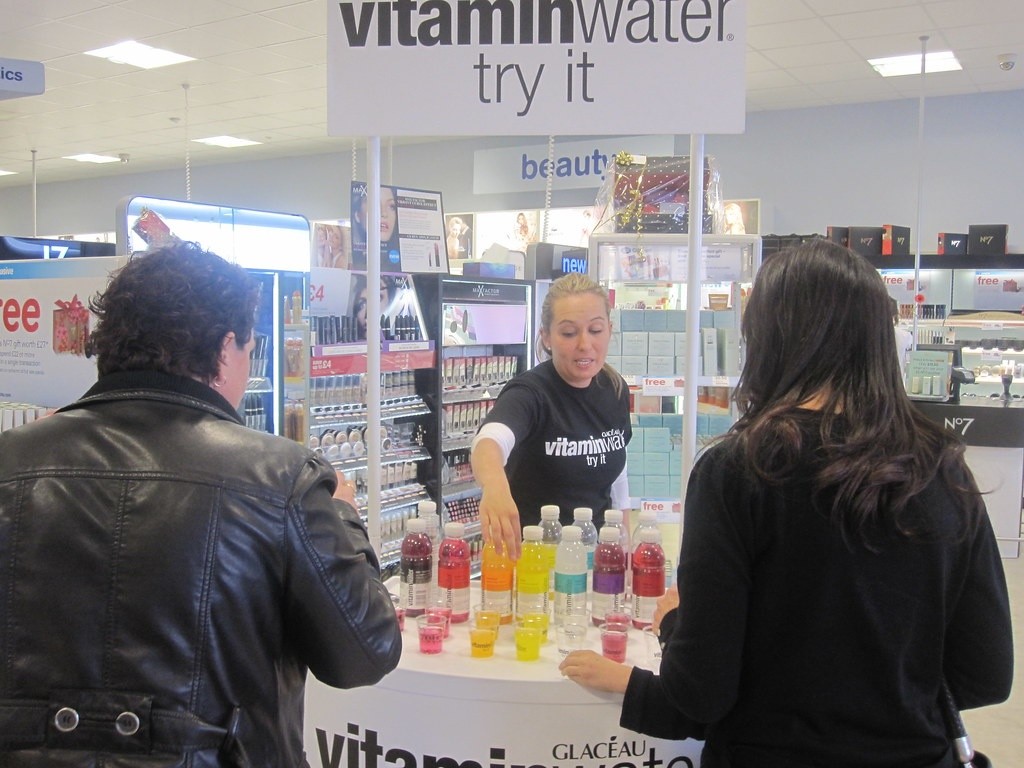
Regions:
[{"x1": 347, "y1": 483, "x2": 356, "y2": 491}]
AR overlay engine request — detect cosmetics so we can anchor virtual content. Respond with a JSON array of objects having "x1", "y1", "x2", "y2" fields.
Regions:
[
  {"x1": 898, "y1": 304, "x2": 1023, "y2": 401},
  {"x1": 240, "y1": 293, "x2": 518, "y2": 577},
  {"x1": 601, "y1": 292, "x2": 747, "y2": 510}
]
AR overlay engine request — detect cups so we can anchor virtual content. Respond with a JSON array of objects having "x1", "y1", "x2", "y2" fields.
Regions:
[{"x1": 389, "y1": 602, "x2": 664, "y2": 664}]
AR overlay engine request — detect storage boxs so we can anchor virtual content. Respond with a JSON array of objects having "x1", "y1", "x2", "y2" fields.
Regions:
[
  {"x1": 912, "y1": 376, "x2": 941, "y2": 395},
  {"x1": 605, "y1": 246, "x2": 740, "y2": 501},
  {"x1": 762, "y1": 224, "x2": 1009, "y2": 260},
  {"x1": 614, "y1": 156, "x2": 713, "y2": 234}
]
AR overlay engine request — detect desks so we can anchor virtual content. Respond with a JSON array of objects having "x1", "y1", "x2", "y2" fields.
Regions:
[{"x1": 301, "y1": 575, "x2": 706, "y2": 768}]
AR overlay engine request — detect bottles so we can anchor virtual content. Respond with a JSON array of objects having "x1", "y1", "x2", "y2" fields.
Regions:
[
  {"x1": 554, "y1": 526, "x2": 587, "y2": 624},
  {"x1": 417, "y1": 501, "x2": 442, "y2": 601},
  {"x1": 439, "y1": 523, "x2": 470, "y2": 622},
  {"x1": 398, "y1": 519, "x2": 433, "y2": 616},
  {"x1": 481, "y1": 524, "x2": 514, "y2": 624},
  {"x1": 592, "y1": 527, "x2": 625, "y2": 626},
  {"x1": 601, "y1": 510, "x2": 628, "y2": 579},
  {"x1": 632, "y1": 512, "x2": 662, "y2": 557},
  {"x1": 632, "y1": 527, "x2": 665, "y2": 630},
  {"x1": 538, "y1": 505, "x2": 562, "y2": 601},
  {"x1": 573, "y1": 507, "x2": 598, "y2": 595},
  {"x1": 517, "y1": 526, "x2": 549, "y2": 626}
]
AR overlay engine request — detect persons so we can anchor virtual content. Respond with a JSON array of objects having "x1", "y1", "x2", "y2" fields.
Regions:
[
  {"x1": 511, "y1": 214, "x2": 529, "y2": 249},
  {"x1": 314, "y1": 227, "x2": 348, "y2": 269},
  {"x1": 350, "y1": 275, "x2": 397, "y2": 344},
  {"x1": 721, "y1": 202, "x2": 747, "y2": 235},
  {"x1": 469, "y1": 273, "x2": 633, "y2": 561},
  {"x1": 446, "y1": 215, "x2": 471, "y2": 259},
  {"x1": 558, "y1": 238, "x2": 1014, "y2": 768},
  {"x1": 351, "y1": 186, "x2": 401, "y2": 271},
  {"x1": 0, "y1": 239, "x2": 403, "y2": 768}
]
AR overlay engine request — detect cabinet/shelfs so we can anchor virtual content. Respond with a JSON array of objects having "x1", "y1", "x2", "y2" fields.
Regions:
[
  {"x1": 235, "y1": 270, "x2": 536, "y2": 581},
  {"x1": 588, "y1": 234, "x2": 763, "y2": 589},
  {"x1": 896, "y1": 319, "x2": 1024, "y2": 384}
]
